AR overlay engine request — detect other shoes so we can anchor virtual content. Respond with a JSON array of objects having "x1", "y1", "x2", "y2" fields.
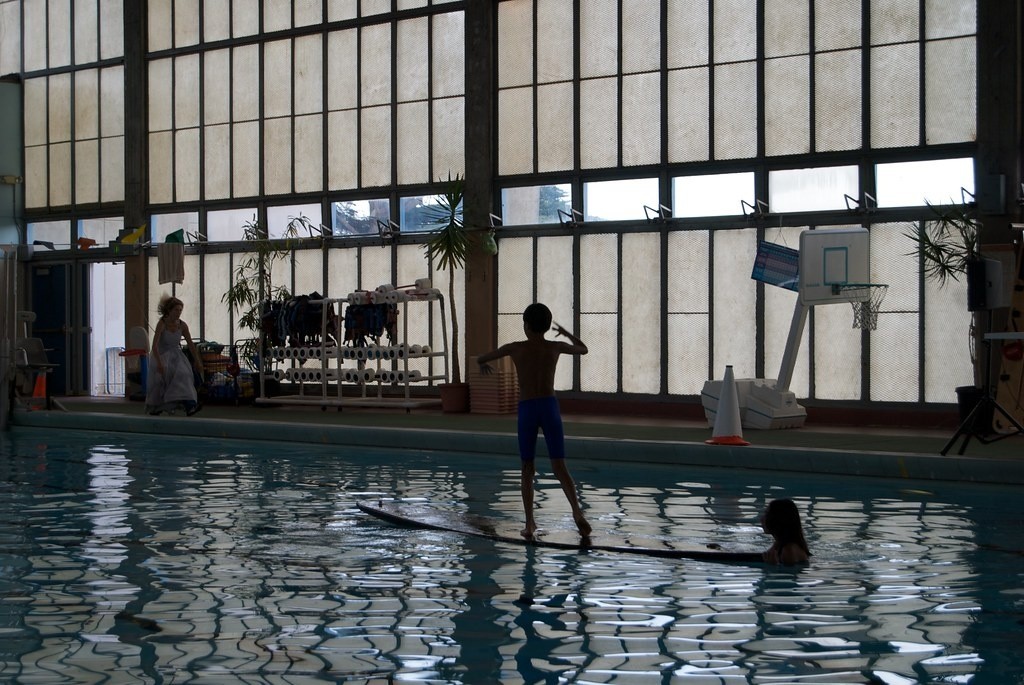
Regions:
[{"x1": 187, "y1": 404, "x2": 200, "y2": 416}]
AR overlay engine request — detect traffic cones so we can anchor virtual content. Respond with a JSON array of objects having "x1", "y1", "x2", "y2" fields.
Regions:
[
  {"x1": 704, "y1": 363, "x2": 751, "y2": 445},
  {"x1": 30, "y1": 370, "x2": 46, "y2": 410}
]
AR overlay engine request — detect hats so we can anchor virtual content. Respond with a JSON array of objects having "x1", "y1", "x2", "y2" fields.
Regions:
[{"x1": 158, "y1": 297, "x2": 184, "y2": 315}]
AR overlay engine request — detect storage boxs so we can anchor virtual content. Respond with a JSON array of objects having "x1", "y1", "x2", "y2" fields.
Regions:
[{"x1": 468, "y1": 355, "x2": 521, "y2": 415}]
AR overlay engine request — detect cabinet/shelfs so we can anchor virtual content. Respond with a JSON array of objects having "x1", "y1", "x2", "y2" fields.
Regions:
[{"x1": 255, "y1": 294, "x2": 449, "y2": 414}]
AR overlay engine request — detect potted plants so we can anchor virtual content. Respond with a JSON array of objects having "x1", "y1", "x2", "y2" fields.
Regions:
[
  {"x1": 220, "y1": 215, "x2": 311, "y2": 408},
  {"x1": 418, "y1": 171, "x2": 496, "y2": 414},
  {"x1": 904, "y1": 187, "x2": 997, "y2": 435}
]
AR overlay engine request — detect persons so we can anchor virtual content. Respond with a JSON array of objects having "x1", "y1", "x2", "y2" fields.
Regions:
[
  {"x1": 758, "y1": 497, "x2": 813, "y2": 570},
  {"x1": 146, "y1": 295, "x2": 204, "y2": 416},
  {"x1": 477, "y1": 301, "x2": 592, "y2": 539}
]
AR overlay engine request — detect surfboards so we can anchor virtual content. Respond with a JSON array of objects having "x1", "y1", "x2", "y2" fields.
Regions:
[{"x1": 354, "y1": 497, "x2": 774, "y2": 560}]
